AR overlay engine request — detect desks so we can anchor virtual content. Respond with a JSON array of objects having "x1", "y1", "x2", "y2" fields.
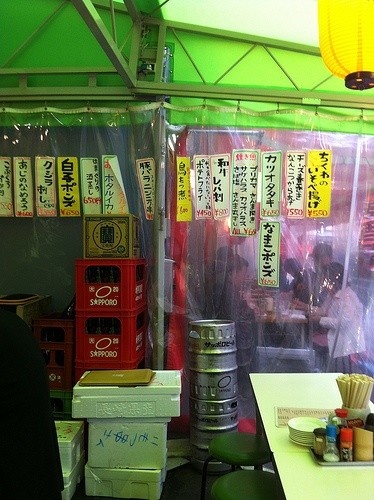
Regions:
[
  {"x1": 249, "y1": 372, "x2": 374, "y2": 500},
  {"x1": 240, "y1": 289, "x2": 311, "y2": 349}
]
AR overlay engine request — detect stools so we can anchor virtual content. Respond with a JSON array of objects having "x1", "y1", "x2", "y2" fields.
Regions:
[
  {"x1": 209, "y1": 469, "x2": 285, "y2": 500},
  {"x1": 200, "y1": 432, "x2": 271, "y2": 500}
]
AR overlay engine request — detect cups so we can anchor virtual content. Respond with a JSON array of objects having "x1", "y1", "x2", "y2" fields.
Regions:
[
  {"x1": 342, "y1": 404, "x2": 371, "y2": 427},
  {"x1": 265, "y1": 297, "x2": 274, "y2": 312}
]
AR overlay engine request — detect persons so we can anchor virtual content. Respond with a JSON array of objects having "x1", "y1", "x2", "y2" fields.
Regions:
[
  {"x1": 201, "y1": 243, "x2": 333, "y2": 373},
  {"x1": 290, "y1": 262, "x2": 365, "y2": 375}
]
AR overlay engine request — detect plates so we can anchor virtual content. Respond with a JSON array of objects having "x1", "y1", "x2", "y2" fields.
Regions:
[{"x1": 287, "y1": 416, "x2": 326, "y2": 447}]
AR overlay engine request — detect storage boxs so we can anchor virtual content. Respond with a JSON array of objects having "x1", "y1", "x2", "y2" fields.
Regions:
[{"x1": 0, "y1": 214, "x2": 182, "y2": 500}]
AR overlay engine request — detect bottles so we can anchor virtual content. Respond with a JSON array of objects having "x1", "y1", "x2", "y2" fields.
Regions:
[
  {"x1": 313, "y1": 427, "x2": 326, "y2": 455},
  {"x1": 334, "y1": 408, "x2": 349, "y2": 450},
  {"x1": 86, "y1": 266, "x2": 118, "y2": 283},
  {"x1": 85, "y1": 318, "x2": 117, "y2": 334},
  {"x1": 339, "y1": 427, "x2": 353, "y2": 462},
  {"x1": 322, "y1": 424, "x2": 340, "y2": 462}
]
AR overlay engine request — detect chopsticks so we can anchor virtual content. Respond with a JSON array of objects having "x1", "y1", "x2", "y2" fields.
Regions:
[{"x1": 335, "y1": 372, "x2": 374, "y2": 409}]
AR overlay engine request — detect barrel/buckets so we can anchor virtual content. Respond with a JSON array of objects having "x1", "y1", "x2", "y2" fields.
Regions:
[{"x1": 188, "y1": 319, "x2": 239, "y2": 473}]
